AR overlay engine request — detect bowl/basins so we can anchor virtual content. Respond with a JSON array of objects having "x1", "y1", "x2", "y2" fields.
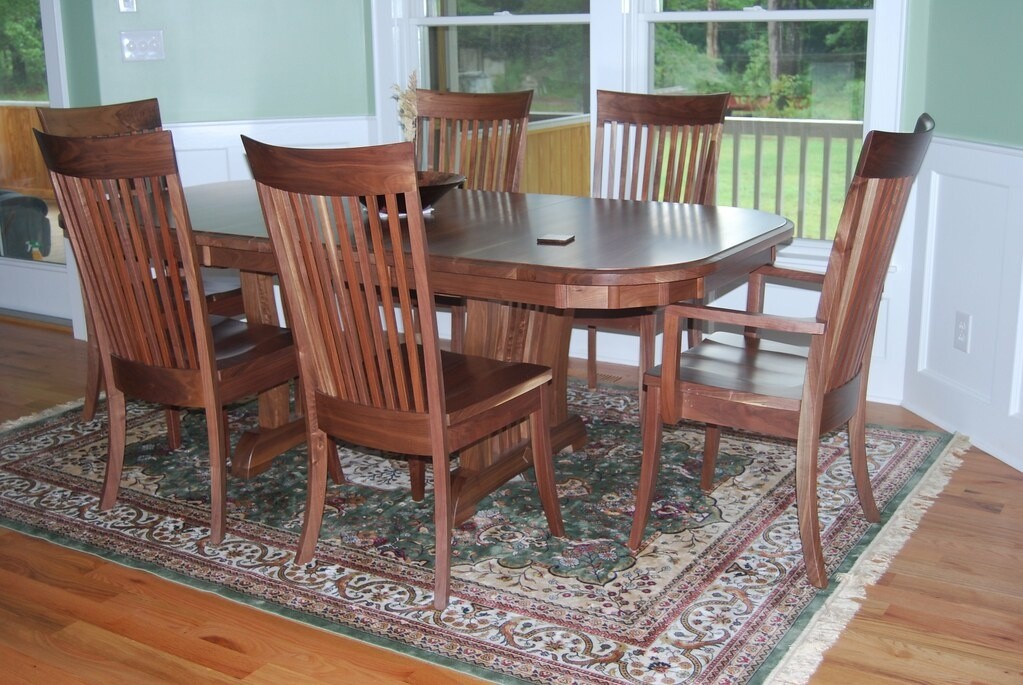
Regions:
[{"x1": 360, "y1": 169, "x2": 466, "y2": 213}]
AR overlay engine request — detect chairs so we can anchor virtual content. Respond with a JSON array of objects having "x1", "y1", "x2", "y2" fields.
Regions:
[
  {"x1": 34, "y1": 97, "x2": 245, "y2": 450},
  {"x1": 240, "y1": 133, "x2": 565, "y2": 609},
  {"x1": 572, "y1": 89, "x2": 735, "y2": 444},
  {"x1": 381, "y1": 89, "x2": 535, "y2": 353},
  {"x1": 30, "y1": 127, "x2": 344, "y2": 545},
  {"x1": 628, "y1": 112, "x2": 936, "y2": 590}
]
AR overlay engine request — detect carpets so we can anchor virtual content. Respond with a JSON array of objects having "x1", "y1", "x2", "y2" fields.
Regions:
[{"x1": 0, "y1": 377, "x2": 973, "y2": 684}]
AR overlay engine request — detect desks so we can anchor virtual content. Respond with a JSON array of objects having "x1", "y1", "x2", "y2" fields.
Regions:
[{"x1": 58, "y1": 179, "x2": 796, "y2": 526}]
